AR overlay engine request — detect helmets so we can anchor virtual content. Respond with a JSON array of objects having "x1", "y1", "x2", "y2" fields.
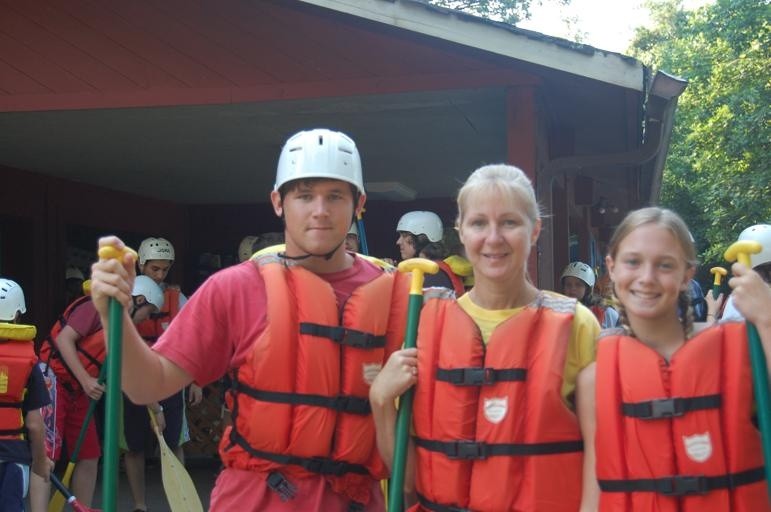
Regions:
[
  {"x1": 273, "y1": 129, "x2": 367, "y2": 200},
  {"x1": 137, "y1": 237, "x2": 177, "y2": 275},
  {"x1": 131, "y1": 276, "x2": 165, "y2": 314},
  {"x1": 0, "y1": 277, "x2": 27, "y2": 322},
  {"x1": 558, "y1": 261, "x2": 595, "y2": 290},
  {"x1": 396, "y1": 210, "x2": 444, "y2": 243},
  {"x1": 239, "y1": 234, "x2": 258, "y2": 264},
  {"x1": 738, "y1": 222, "x2": 771, "y2": 271}
]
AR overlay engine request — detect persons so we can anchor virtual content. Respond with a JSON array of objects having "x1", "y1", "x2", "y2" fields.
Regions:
[
  {"x1": 90, "y1": 126, "x2": 412, "y2": 510},
  {"x1": 346, "y1": 210, "x2": 473, "y2": 309},
  {"x1": 0, "y1": 277, "x2": 57, "y2": 512},
  {"x1": 119, "y1": 236, "x2": 204, "y2": 511},
  {"x1": 31, "y1": 274, "x2": 166, "y2": 512},
  {"x1": 239, "y1": 237, "x2": 267, "y2": 262},
  {"x1": 561, "y1": 262, "x2": 620, "y2": 330},
  {"x1": 368, "y1": 158, "x2": 603, "y2": 511},
  {"x1": 576, "y1": 204, "x2": 771, "y2": 509},
  {"x1": 690, "y1": 224, "x2": 770, "y2": 326}
]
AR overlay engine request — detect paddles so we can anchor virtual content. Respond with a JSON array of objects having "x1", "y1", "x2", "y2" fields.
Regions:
[
  {"x1": 145, "y1": 405, "x2": 204, "y2": 512},
  {"x1": 48, "y1": 356, "x2": 105, "y2": 510}
]
